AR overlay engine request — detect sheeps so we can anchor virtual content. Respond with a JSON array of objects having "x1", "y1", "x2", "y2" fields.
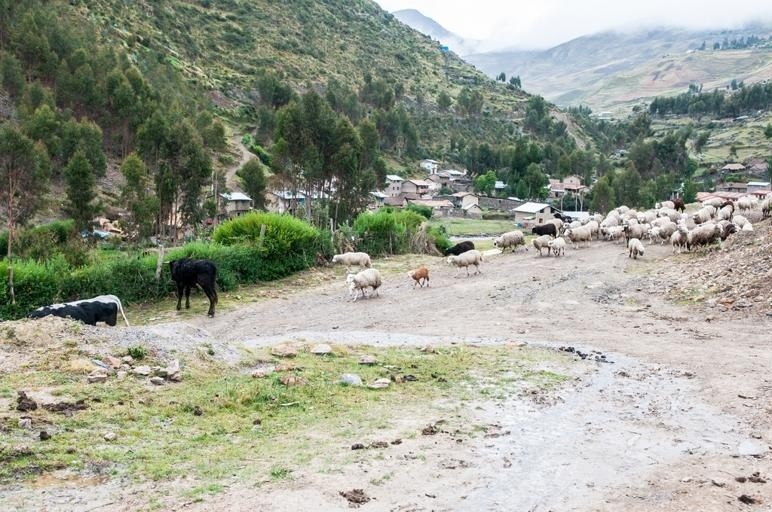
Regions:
[
  {"x1": 446, "y1": 250, "x2": 483, "y2": 278},
  {"x1": 408, "y1": 265, "x2": 430, "y2": 289},
  {"x1": 444, "y1": 241, "x2": 475, "y2": 256},
  {"x1": 346, "y1": 268, "x2": 382, "y2": 302},
  {"x1": 531, "y1": 193, "x2": 772, "y2": 260},
  {"x1": 331, "y1": 251, "x2": 372, "y2": 274},
  {"x1": 493, "y1": 230, "x2": 528, "y2": 254}
]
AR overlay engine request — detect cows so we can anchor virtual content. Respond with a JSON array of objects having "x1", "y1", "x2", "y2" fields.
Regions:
[
  {"x1": 26, "y1": 294, "x2": 129, "y2": 328},
  {"x1": 161, "y1": 254, "x2": 219, "y2": 318}
]
